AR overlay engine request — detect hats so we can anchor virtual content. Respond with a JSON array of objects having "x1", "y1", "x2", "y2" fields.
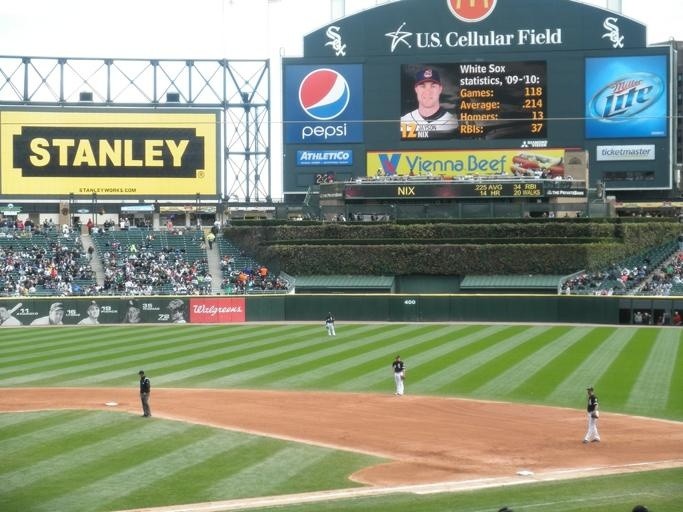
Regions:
[
  {"x1": 49, "y1": 303, "x2": 63, "y2": 311},
  {"x1": 414, "y1": 69, "x2": 439, "y2": 86},
  {"x1": 86, "y1": 300, "x2": 98, "y2": 310},
  {"x1": 166, "y1": 299, "x2": 183, "y2": 310},
  {"x1": 586, "y1": 387, "x2": 593, "y2": 392},
  {"x1": 127, "y1": 299, "x2": 138, "y2": 310}
]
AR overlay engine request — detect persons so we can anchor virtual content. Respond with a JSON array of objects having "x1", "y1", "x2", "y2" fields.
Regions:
[
  {"x1": 78, "y1": 300, "x2": 100, "y2": 325},
  {"x1": 138, "y1": 371, "x2": 151, "y2": 417},
  {"x1": 523, "y1": 197, "x2": 681, "y2": 217},
  {"x1": 122, "y1": 299, "x2": 144, "y2": 323},
  {"x1": 401, "y1": 70, "x2": 457, "y2": 132},
  {"x1": 168, "y1": 299, "x2": 188, "y2": 324},
  {"x1": 634, "y1": 311, "x2": 681, "y2": 325},
  {"x1": 583, "y1": 387, "x2": 600, "y2": 443},
  {"x1": 2, "y1": 304, "x2": 23, "y2": 325},
  {"x1": 327, "y1": 169, "x2": 575, "y2": 188},
  {"x1": 326, "y1": 312, "x2": 336, "y2": 336},
  {"x1": 2, "y1": 215, "x2": 290, "y2": 297},
  {"x1": 560, "y1": 234, "x2": 681, "y2": 296},
  {"x1": 30, "y1": 302, "x2": 65, "y2": 325},
  {"x1": 293, "y1": 211, "x2": 387, "y2": 221},
  {"x1": 392, "y1": 356, "x2": 405, "y2": 395}
]
